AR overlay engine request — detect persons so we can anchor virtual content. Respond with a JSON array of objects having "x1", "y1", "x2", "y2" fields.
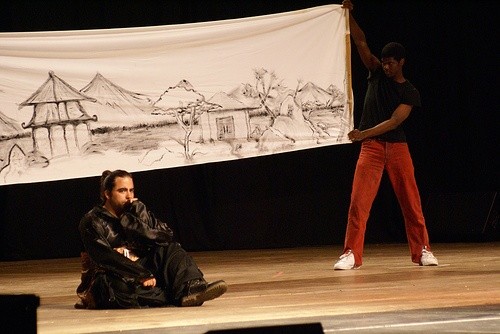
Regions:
[
  {"x1": 76, "y1": 169, "x2": 227, "y2": 310},
  {"x1": 334, "y1": 0, "x2": 438, "y2": 270}
]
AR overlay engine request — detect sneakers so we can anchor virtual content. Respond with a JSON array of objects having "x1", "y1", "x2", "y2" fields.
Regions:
[
  {"x1": 334, "y1": 250, "x2": 361, "y2": 270},
  {"x1": 183, "y1": 280, "x2": 228, "y2": 307},
  {"x1": 419, "y1": 249, "x2": 438, "y2": 266}
]
